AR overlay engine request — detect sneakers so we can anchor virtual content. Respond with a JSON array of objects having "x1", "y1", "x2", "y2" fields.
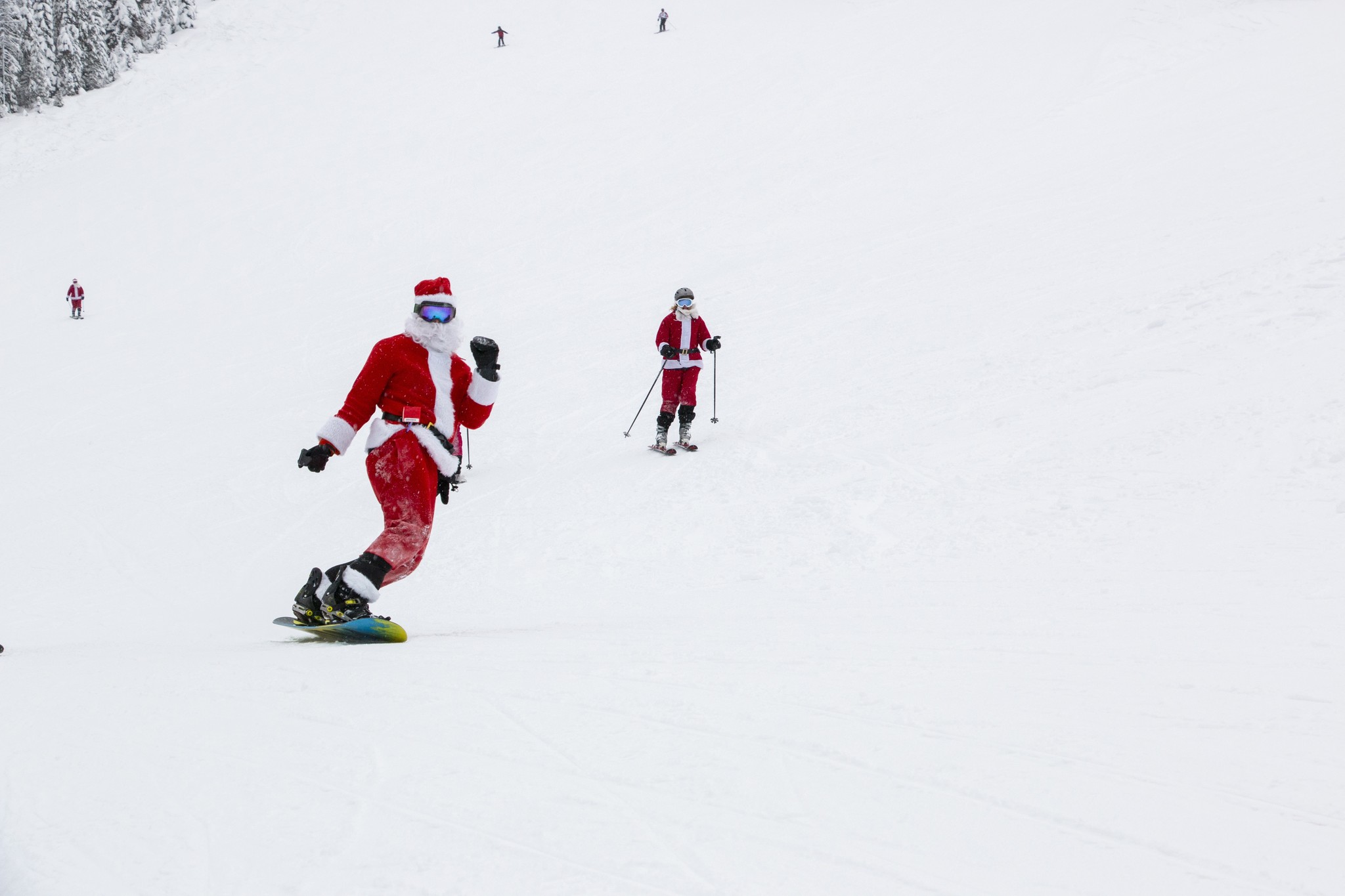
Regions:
[
  {"x1": 320, "y1": 563, "x2": 391, "y2": 623},
  {"x1": 679, "y1": 422, "x2": 692, "y2": 442},
  {"x1": 292, "y1": 567, "x2": 326, "y2": 626},
  {"x1": 656, "y1": 425, "x2": 668, "y2": 446}
]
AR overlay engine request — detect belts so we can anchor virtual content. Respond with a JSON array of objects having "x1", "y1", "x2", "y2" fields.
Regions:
[
  {"x1": 672, "y1": 347, "x2": 699, "y2": 355},
  {"x1": 382, "y1": 412, "x2": 435, "y2": 433}
]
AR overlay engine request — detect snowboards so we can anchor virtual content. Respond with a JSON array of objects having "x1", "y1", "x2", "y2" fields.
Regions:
[{"x1": 272, "y1": 617, "x2": 408, "y2": 643}]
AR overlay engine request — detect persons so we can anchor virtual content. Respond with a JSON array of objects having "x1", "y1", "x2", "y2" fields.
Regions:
[
  {"x1": 491, "y1": 26, "x2": 508, "y2": 47},
  {"x1": 67, "y1": 279, "x2": 85, "y2": 317},
  {"x1": 290, "y1": 279, "x2": 501, "y2": 626},
  {"x1": 656, "y1": 287, "x2": 721, "y2": 450},
  {"x1": 658, "y1": 8, "x2": 669, "y2": 32}
]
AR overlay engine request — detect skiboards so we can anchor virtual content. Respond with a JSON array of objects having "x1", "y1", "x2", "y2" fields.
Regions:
[
  {"x1": 654, "y1": 29, "x2": 669, "y2": 34},
  {"x1": 494, "y1": 44, "x2": 509, "y2": 48},
  {"x1": 647, "y1": 442, "x2": 698, "y2": 455},
  {"x1": 69, "y1": 316, "x2": 84, "y2": 320}
]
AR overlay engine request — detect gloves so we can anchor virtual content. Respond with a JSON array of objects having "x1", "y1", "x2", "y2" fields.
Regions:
[
  {"x1": 81, "y1": 296, "x2": 84, "y2": 300},
  {"x1": 66, "y1": 297, "x2": 68, "y2": 301},
  {"x1": 660, "y1": 345, "x2": 675, "y2": 359},
  {"x1": 470, "y1": 336, "x2": 500, "y2": 382},
  {"x1": 299, "y1": 445, "x2": 331, "y2": 473},
  {"x1": 706, "y1": 339, "x2": 721, "y2": 351}
]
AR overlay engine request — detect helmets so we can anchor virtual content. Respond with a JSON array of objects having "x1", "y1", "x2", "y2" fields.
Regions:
[{"x1": 674, "y1": 288, "x2": 694, "y2": 301}]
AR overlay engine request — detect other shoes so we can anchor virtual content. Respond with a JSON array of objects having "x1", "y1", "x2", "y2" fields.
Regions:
[
  {"x1": 72, "y1": 311, "x2": 75, "y2": 317},
  {"x1": 78, "y1": 311, "x2": 81, "y2": 317}
]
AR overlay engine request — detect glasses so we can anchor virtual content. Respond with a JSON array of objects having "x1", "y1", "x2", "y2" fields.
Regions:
[
  {"x1": 419, "y1": 303, "x2": 456, "y2": 323},
  {"x1": 677, "y1": 298, "x2": 693, "y2": 308}
]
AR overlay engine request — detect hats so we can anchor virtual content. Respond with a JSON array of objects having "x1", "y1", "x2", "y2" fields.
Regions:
[
  {"x1": 414, "y1": 277, "x2": 454, "y2": 304},
  {"x1": 72, "y1": 279, "x2": 77, "y2": 281}
]
